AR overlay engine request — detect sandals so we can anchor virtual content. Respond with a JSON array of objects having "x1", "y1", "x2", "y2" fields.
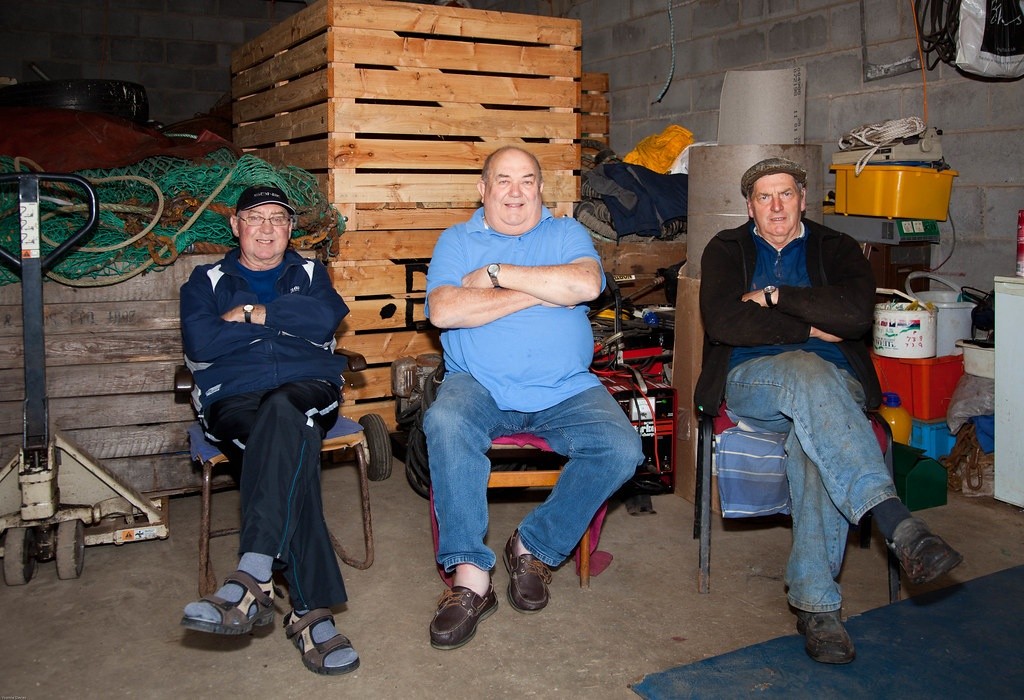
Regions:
[
  {"x1": 282, "y1": 607, "x2": 361, "y2": 676},
  {"x1": 182, "y1": 569, "x2": 277, "y2": 634}
]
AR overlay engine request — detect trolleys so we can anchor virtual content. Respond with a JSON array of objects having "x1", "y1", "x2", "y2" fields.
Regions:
[{"x1": 0, "y1": 173, "x2": 172, "y2": 588}]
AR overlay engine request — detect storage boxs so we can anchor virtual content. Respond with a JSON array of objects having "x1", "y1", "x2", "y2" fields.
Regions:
[
  {"x1": 830, "y1": 164, "x2": 998, "y2": 474},
  {"x1": 0, "y1": 0, "x2": 611, "y2": 496}
]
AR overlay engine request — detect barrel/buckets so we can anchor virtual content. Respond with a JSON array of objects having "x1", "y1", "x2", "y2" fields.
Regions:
[
  {"x1": 872, "y1": 287, "x2": 938, "y2": 359},
  {"x1": 905, "y1": 270, "x2": 977, "y2": 357}
]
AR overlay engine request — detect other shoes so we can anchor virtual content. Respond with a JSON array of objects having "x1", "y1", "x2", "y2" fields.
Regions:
[
  {"x1": 887, "y1": 522, "x2": 961, "y2": 585},
  {"x1": 798, "y1": 603, "x2": 853, "y2": 664}
]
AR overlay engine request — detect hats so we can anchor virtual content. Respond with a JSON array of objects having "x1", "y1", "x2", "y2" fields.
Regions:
[
  {"x1": 741, "y1": 156, "x2": 808, "y2": 202},
  {"x1": 236, "y1": 185, "x2": 297, "y2": 217}
]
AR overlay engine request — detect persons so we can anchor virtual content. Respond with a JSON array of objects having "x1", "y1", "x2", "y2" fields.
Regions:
[
  {"x1": 693, "y1": 157, "x2": 964, "y2": 666},
  {"x1": 178, "y1": 183, "x2": 360, "y2": 678},
  {"x1": 421, "y1": 146, "x2": 646, "y2": 650}
]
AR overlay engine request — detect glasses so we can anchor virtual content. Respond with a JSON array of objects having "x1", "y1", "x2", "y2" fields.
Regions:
[{"x1": 236, "y1": 214, "x2": 292, "y2": 226}]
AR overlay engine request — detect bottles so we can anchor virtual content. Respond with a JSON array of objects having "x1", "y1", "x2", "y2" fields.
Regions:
[
  {"x1": 642, "y1": 308, "x2": 658, "y2": 326},
  {"x1": 878, "y1": 391, "x2": 912, "y2": 446}
]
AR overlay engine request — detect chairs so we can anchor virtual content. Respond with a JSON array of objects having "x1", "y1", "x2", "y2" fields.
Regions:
[
  {"x1": 433, "y1": 433, "x2": 617, "y2": 589},
  {"x1": 693, "y1": 402, "x2": 900, "y2": 600},
  {"x1": 174, "y1": 347, "x2": 376, "y2": 603}
]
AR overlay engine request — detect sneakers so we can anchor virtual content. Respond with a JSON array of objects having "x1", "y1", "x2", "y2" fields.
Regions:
[
  {"x1": 504, "y1": 526, "x2": 551, "y2": 616},
  {"x1": 429, "y1": 582, "x2": 498, "y2": 650}
]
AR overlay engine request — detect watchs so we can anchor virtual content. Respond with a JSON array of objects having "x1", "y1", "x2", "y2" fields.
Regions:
[
  {"x1": 243, "y1": 303, "x2": 255, "y2": 325},
  {"x1": 763, "y1": 285, "x2": 777, "y2": 307},
  {"x1": 487, "y1": 262, "x2": 502, "y2": 288}
]
